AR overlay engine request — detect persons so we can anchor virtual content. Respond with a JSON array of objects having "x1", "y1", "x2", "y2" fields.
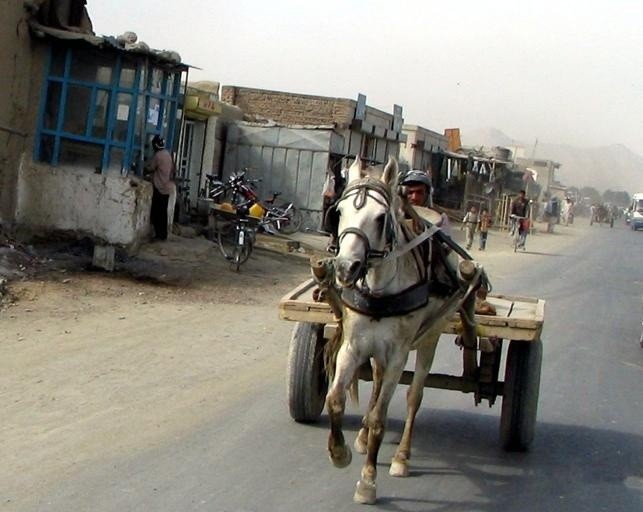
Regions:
[
  {"x1": 398, "y1": 169, "x2": 497, "y2": 315},
  {"x1": 134, "y1": 134, "x2": 175, "y2": 244},
  {"x1": 508, "y1": 189, "x2": 530, "y2": 246},
  {"x1": 460, "y1": 206, "x2": 480, "y2": 250},
  {"x1": 475, "y1": 207, "x2": 493, "y2": 251},
  {"x1": 543, "y1": 195, "x2": 618, "y2": 233}
]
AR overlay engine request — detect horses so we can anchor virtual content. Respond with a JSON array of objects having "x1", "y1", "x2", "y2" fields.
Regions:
[{"x1": 323, "y1": 153, "x2": 459, "y2": 506}]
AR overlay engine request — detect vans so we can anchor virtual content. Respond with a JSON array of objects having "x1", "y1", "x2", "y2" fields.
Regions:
[{"x1": 630, "y1": 193, "x2": 643, "y2": 231}]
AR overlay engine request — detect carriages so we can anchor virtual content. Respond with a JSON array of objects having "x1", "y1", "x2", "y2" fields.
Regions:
[
  {"x1": 276, "y1": 152, "x2": 546, "y2": 506},
  {"x1": 588, "y1": 203, "x2": 614, "y2": 228}
]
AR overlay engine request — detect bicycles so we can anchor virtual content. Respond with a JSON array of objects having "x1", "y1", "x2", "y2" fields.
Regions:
[
  {"x1": 509, "y1": 215, "x2": 527, "y2": 253},
  {"x1": 195, "y1": 166, "x2": 302, "y2": 272}
]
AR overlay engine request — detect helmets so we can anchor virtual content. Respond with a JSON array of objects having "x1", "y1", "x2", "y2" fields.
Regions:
[{"x1": 398, "y1": 169, "x2": 431, "y2": 192}]
